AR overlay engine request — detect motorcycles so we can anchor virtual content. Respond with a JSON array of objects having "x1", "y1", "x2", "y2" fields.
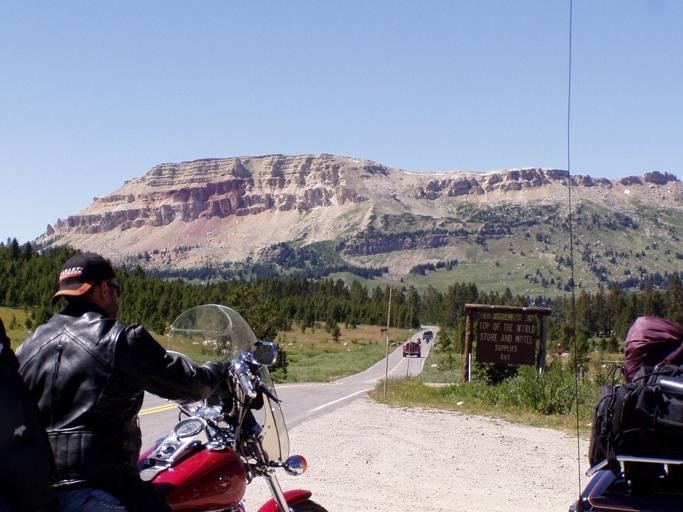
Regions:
[{"x1": 135, "y1": 301, "x2": 332, "y2": 512}]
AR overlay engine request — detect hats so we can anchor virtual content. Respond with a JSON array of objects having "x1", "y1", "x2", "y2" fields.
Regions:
[{"x1": 50, "y1": 253, "x2": 115, "y2": 308}]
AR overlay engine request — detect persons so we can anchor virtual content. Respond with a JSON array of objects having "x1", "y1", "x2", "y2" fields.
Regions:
[
  {"x1": 569, "y1": 316, "x2": 683, "y2": 512},
  {"x1": 0, "y1": 319, "x2": 58, "y2": 512},
  {"x1": 14, "y1": 254, "x2": 228, "y2": 512}
]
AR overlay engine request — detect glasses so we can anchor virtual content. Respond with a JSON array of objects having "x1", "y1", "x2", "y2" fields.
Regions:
[{"x1": 97, "y1": 279, "x2": 121, "y2": 298}]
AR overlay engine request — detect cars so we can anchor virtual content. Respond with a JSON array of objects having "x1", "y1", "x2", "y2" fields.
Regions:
[
  {"x1": 403, "y1": 341, "x2": 420, "y2": 357},
  {"x1": 423, "y1": 331, "x2": 433, "y2": 340}
]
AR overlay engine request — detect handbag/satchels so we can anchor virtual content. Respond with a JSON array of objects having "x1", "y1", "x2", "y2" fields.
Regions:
[{"x1": 589, "y1": 315, "x2": 683, "y2": 496}]
